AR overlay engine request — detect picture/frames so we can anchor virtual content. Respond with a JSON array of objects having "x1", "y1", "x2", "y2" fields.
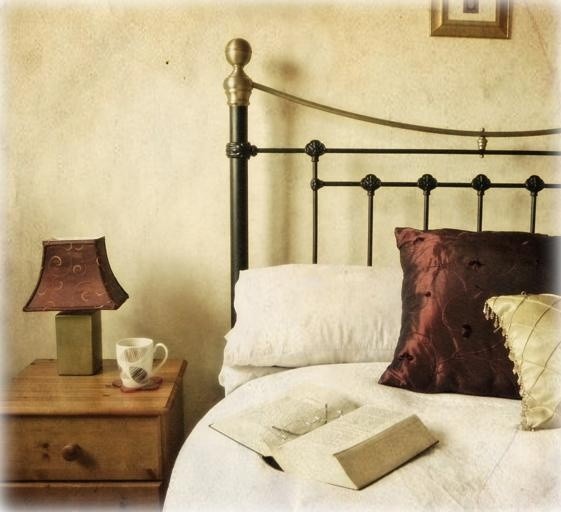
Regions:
[{"x1": 430, "y1": 0, "x2": 514, "y2": 39}]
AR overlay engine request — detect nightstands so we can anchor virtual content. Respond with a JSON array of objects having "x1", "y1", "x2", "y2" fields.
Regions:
[{"x1": 1, "y1": 359, "x2": 188, "y2": 512}]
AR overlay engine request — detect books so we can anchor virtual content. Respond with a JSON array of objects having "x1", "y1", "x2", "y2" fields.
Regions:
[{"x1": 208, "y1": 381, "x2": 438, "y2": 492}]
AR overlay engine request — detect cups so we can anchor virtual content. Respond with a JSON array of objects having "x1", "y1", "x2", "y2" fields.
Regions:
[{"x1": 115, "y1": 337, "x2": 168, "y2": 389}]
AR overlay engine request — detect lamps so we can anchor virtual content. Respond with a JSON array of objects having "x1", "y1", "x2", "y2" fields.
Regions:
[{"x1": 22, "y1": 237, "x2": 129, "y2": 376}]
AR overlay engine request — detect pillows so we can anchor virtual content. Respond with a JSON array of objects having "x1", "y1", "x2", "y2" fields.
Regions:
[
  {"x1": 380, "y1": 226, "x2": 561, "y2": 394},
  {"x1": 224, "y1": 262, "x2": 403, "y2": 366},
  {"x1": 483, "y1": 293, "x2": 561, "y2": 429}
]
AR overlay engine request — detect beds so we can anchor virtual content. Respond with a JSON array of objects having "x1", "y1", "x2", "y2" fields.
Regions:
[{"x1": 163, "y1": 38, "x2": 561, "y2": 512}]
